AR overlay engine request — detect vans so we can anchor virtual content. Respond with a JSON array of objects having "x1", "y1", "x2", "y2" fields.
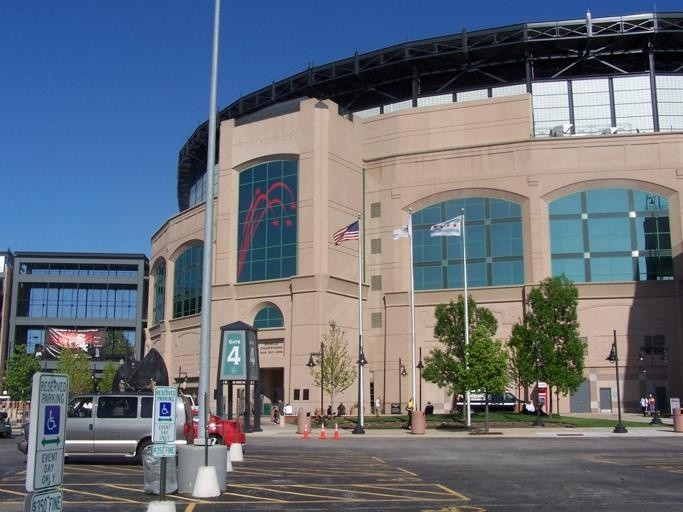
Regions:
[{"x1": 19, "y1": 393, "x2": 194, "y2": 466}]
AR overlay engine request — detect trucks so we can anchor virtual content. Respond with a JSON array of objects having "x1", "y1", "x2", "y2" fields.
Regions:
[{"x1": 452, "y1": 385, "x2": 531, "y2": 414}]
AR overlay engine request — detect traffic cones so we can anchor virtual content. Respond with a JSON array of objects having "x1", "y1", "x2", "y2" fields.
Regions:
[
  {"x1": 331, "y1": 422, "x2": 342, "y2": 441},
  {"x1": 318, "y1": 422, "x2": 327, "y2": 440},
  {"x1": 300, "y1": 422, "x2": 310, "y2": 440}
]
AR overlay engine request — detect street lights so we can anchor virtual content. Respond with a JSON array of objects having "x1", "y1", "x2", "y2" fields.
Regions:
[
  {"x1": 416, "y1": 344, "x2": 424, "y2": 411},
  {"x1": 638, "y1": 330, "x2": 662, "y2": 425},
  {"x1": 5, "y1": 335, "x2": 40, "y2": 389},
  {"x1": 90, "y1": 363, "x2": 97, "y2": 392},
  {"x1": 606, "y1": 330, "x2": 629, "y2": 434},
  {"x1": 350, "y1": 334, "x2": 370, "y2": 434},
  {"x1": 658, "y1": 340, "x2": 671, "y2": 418},
  {"x1": 395, "y1": 357, "x2": 408, "y2": 414},
  {"x1": 305, "y1": 341, "x2": 326, "y2": 426},
  {"x1": 529, "y1": 338, "x2": 545, "y2": 427}
]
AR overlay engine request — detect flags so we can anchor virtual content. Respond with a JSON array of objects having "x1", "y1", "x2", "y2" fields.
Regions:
[
  {"x1": 429, "y1": 216, "x2": 461, "y2": 238},
  {"x1": 392, "y1": 224, "x2": 409, "y2": 239},
  {"x1": 331, "y1": 220, "x2": 359, "y2": 246}
]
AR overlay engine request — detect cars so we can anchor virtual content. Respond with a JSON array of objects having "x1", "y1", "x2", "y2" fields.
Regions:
[
  {"x1": 182, "y1": 393, "x2": 199, "y2": 412},
  {"x1": 0, "y1": 411, "x2": 13, "y2": 439},
  {"x1": 191, "y1": 406, "x2": 245, "y2": 454},
  {"x1": 390, "y1": 404, "x2": 399, "y2": 413}
]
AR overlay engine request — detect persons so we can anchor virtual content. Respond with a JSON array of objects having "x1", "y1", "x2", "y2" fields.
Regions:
[
  {"x1": 406, "y1": 406, "x2": 413, "y2": 429},
  {"x1": 648, "y1": 393, "x2": 656, "y2": 411},
  {"x1": 268, "y1": 397, "x2": 348, "y2": 426},
  {"x1": 406, "y1": 398, "x2": 413, "y2": 410},
  {"x1": 639, "y1": 394, "x2": 649, "y2": 416},
  {"x1": 424, "y1": 401, "x2": 433, "y2": 414},
  {"x1": 374, "y1": 396, "x2": 381, "y2": 417},
  {"x1": 525, "y1": 400, "x2": 535, "y2": 413}
]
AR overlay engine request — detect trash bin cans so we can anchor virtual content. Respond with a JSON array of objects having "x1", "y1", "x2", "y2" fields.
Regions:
[
  {"x1": 298, "y1": 407, "x2": 312, "y2": 434},
  {"x1": 141, "y1": 445, "x2": 178, "y2": 494},
  {"x1": 673, "y1": 408, "x2": 683, "y2": 432}
]
